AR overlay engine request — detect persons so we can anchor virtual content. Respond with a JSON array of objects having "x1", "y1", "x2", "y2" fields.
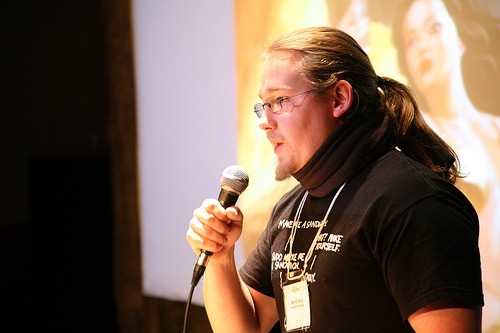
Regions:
[
  {"x1": 392, "y1": 0, "x2": 500, "y2": 333},
  {"x1": 186, "y1": 27, "x2": 485, "y2": 333}
]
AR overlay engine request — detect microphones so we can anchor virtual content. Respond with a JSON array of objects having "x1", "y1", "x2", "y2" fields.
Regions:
[{"x1": 191, "y1": 165, "x2": 249, "y2": 286}]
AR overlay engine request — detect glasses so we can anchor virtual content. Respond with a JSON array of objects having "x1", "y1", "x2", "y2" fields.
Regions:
[{"x1": 252, "y1": 87, "x2": 318, "y2": 117}]
